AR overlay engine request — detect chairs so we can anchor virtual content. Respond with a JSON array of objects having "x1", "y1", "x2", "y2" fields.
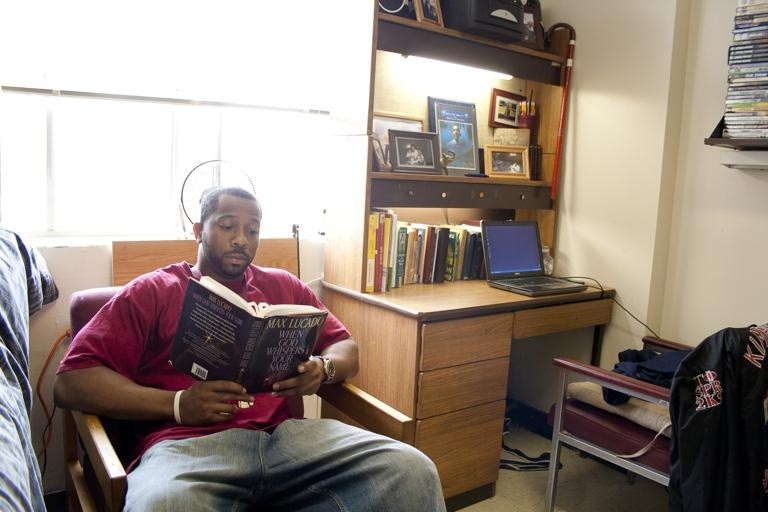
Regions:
[
  {"x1": 543, "y1": 324, "x2": 767, "y2": 512},
  {"x1": 62, "y1": 238, "x2": 414, "y2": 512}
]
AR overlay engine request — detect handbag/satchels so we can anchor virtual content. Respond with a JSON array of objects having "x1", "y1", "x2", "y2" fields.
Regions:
[{"x1": 618, "y1": 347, "x2": 693, "y2": 388}]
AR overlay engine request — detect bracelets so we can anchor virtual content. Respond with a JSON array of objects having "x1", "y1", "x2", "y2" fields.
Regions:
[{"x1": 173, "y1": 388, "x2": 187, "y2": 426}]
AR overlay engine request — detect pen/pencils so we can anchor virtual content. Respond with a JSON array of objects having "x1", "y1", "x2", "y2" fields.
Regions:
[{"x1": 463, "y1": 173, "x2": 490, "y2": 177}]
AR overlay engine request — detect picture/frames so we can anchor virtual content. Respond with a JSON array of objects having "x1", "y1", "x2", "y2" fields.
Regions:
[
  {"x1": 414, "y1": 0, "x2": 444, "y2": 27},
  {"x1": 372, "y1": 84, "x2": 531, "y2": 181}
]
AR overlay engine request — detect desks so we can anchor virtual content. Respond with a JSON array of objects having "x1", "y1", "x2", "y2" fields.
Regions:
[{"x1": 321, "y1": 281, "x2": 616, "y2": 510}]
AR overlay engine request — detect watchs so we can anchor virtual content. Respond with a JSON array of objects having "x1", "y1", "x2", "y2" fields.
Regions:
[{"x1": 315, "y1": 354, "x2": 336, "y2": 387}]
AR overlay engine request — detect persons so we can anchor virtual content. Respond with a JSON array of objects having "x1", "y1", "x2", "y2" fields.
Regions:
[
  {"x1": 504, "y1": 103, "x2": 511, "y2": 120},
  {"x1": 52, "y1": 186, "x2": 447, "y2": 511},
  {"x1": 404, "y1": 143, "x2": 427, "y2": 166},
  {"x1": 445, "y1": 123, "x2": 466, "y2": 168}
]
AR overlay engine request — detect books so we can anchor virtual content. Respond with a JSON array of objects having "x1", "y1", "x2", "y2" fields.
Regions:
[
  {"x1": 723, "y1": 0, "x2": 768, "y2": 140},
  {"x1": 493, "y1": 127, "x2": 541, "y2": 181},
  {"x1": 166, "y1": 273, "x2": 328, "y2": 397},
  {"x1": 428, "y1": 96, "x2": 480, "y2": 177},
  {"x1": 365, "y1": 207, "x2": 487, "y2": 295}
]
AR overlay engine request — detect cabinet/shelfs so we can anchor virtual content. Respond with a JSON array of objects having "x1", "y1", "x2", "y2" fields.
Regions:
[
  {"x1": 413, "y1": 311, "x2": 513, "y2": 512},
  {"x1": 321, "y1": 0, "x2": 577, "y2": 294},
  {"x1": 513, "y1": 300, "x2": 612, "y2": 340}
]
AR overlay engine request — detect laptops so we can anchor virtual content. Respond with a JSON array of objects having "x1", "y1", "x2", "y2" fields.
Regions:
[{"x1": 480, "y1": 219, "x2": 587, "y2": 297}]
